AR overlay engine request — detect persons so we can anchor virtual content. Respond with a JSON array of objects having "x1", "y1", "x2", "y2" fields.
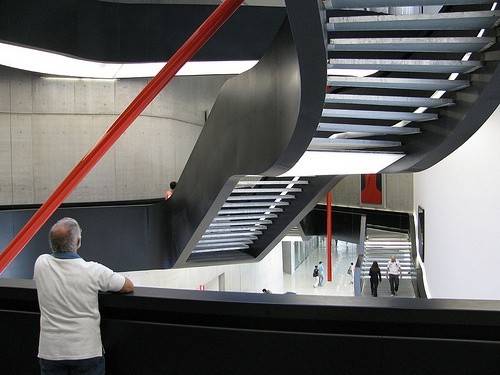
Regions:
[
  {"x1": 34, "y1": 217, "x2": 134, "y2": 375},
  {"x1": 369, "y1": 261, "x2": 381, "y2": 297},
  {"x1": 386, "y1": 255, "x2": 402, "y2": 297},
  {"x1": 313, "y1": 266, "x2": 319, "y2": 288},
  {"x1": 349, "y1": 262, "x2": 354, "y2": 284},
  {"x1": 262, "y1": 289, "x2": 272, "y2": 293},
  {"x1": 165, "y1": 181, "x2": 177, "y2": 200},
  {"x1": 317, "y1": 261, "x2": 324, "y2": 287}
]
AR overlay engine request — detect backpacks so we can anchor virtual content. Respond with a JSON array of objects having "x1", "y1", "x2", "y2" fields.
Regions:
[{"x1": 313, "y1": 271, "x2": 317, "y2": 277}]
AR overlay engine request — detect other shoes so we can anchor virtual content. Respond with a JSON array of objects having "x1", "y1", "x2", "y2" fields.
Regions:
[
  {"x1": 391, "y1": 294, "x2": 394, "y2": 296},
  {"x1": 395, "y1": 291, "x2": 398, "y2": 294}
]
